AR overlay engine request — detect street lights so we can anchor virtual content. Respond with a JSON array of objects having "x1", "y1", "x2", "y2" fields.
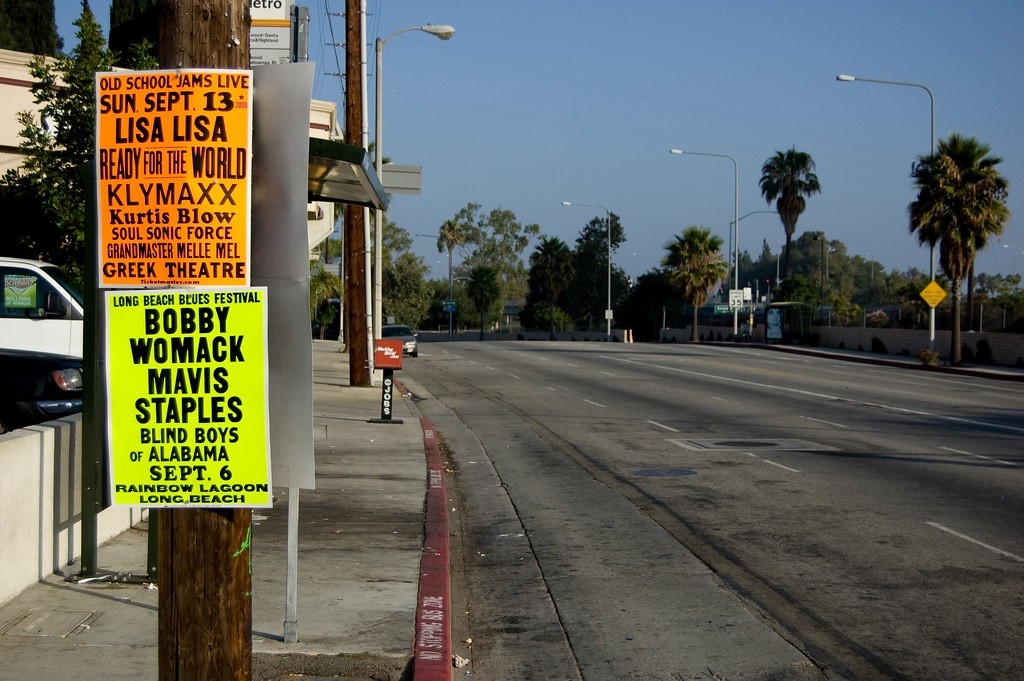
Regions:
[
  {"x1": 373, "y1": 24, "x2": 456, "y2": 340},
  {"x1": 449, "y1": 276, "x2": 474, "y2": 336},
  {"x1": 670, "y1": 148, "x2": 739, "y2": 340},
  {"x1": 1002, "y1": 244, "x2": 1024, "y2": 255},
  {"x1": 562, "y1": 201, "x2": 611, "y2": 341},
  {"x1": 837, "y1": 74, "x2": 935, "y2": 351},
  {"x1": 415, "y1": 233, "x2": 453, "y2": 335}
]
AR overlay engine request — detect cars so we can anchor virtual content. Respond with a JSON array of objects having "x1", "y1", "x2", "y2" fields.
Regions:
[
  {"x1": 0, "y1": 257, "x2": 84, "y2": 357},
  {"x1": 0, "y1": 348, "x2": 83, "y2": 430},
  {"x1": 382, "y1": 325, "x2": 418, "y2": 357}
]
